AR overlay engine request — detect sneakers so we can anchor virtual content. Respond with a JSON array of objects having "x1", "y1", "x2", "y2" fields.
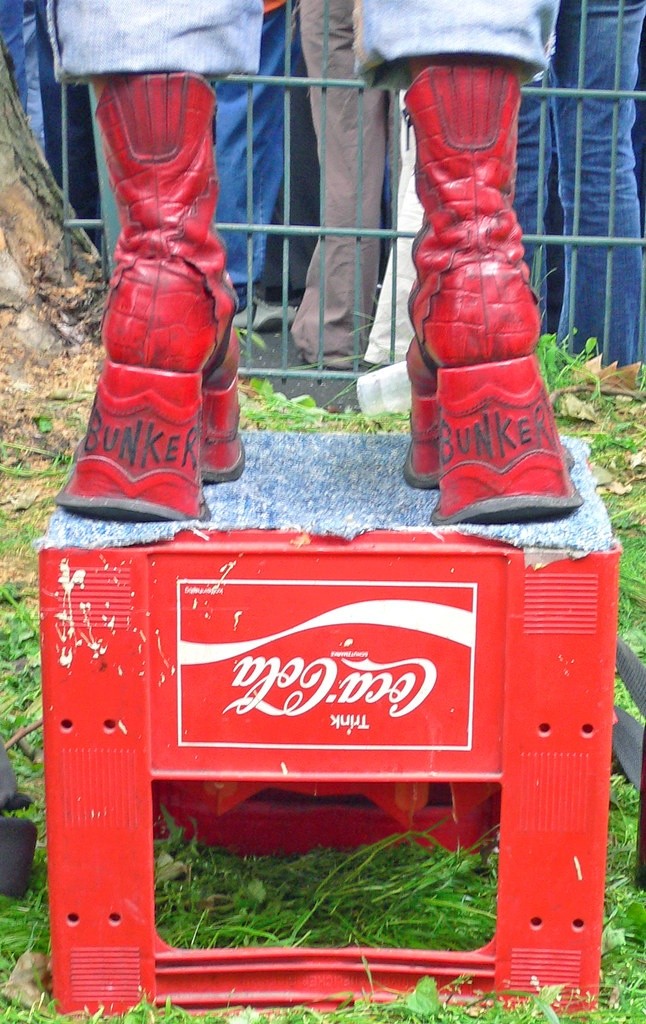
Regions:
[{"x1": 230, "y1": 294, "x2": 298, "y2": 334}]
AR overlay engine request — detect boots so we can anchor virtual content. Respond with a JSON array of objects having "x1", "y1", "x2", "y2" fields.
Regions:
[
  {"x1": 398, "y1": 62, "x2": 586, "y2": 523},
  {"x1": 56, "y1": 75, "x2": 245, "y2": 522}
]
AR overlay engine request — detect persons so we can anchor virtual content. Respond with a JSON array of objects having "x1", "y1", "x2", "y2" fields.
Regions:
[
  {"x1": 0, "y1": 1, "x2": 645, "y2": 391},
  {"x1": 59, "y1": 0, "x2": 585, "y2": 527}
]
